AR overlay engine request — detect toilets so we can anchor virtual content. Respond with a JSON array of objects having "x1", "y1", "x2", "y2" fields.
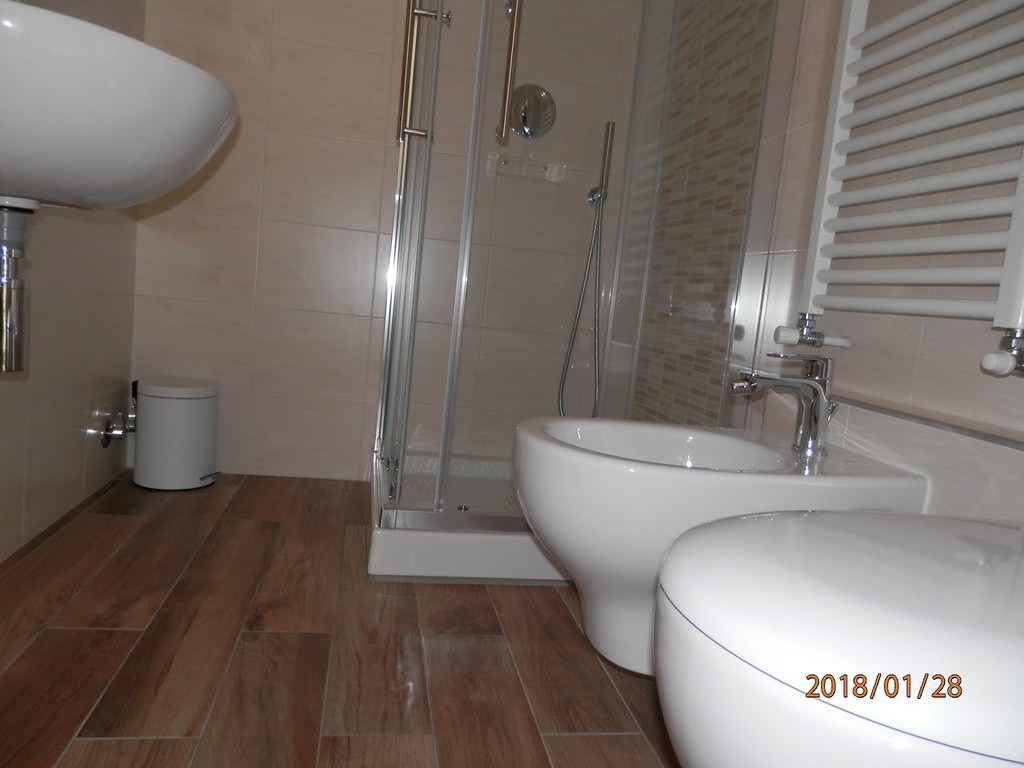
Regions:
[{"x1": 651, "y1": 510, "x2": 1024, "y2": 768}]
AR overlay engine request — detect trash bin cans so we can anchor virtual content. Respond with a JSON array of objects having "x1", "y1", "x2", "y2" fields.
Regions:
[{"x1": 131, "y1": 377, "x2": 222, "y2": 490}]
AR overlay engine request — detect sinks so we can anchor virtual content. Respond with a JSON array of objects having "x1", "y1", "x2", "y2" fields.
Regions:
[
  {"x1": 511, "y1": 416, "x2": 931, "y2": 680},
  {"x1": 0, "y1": 0, "x2": 240, "y2": 214}
]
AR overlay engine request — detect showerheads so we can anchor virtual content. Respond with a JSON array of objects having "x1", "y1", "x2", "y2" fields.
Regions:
[{"x1": 591, "y1": 185, "x2": 607, "y2": 238}]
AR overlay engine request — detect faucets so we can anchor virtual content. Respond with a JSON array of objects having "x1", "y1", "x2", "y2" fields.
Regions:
[{"x1": 729, "y1": 350, "x2": 842, "y2": 456}]
